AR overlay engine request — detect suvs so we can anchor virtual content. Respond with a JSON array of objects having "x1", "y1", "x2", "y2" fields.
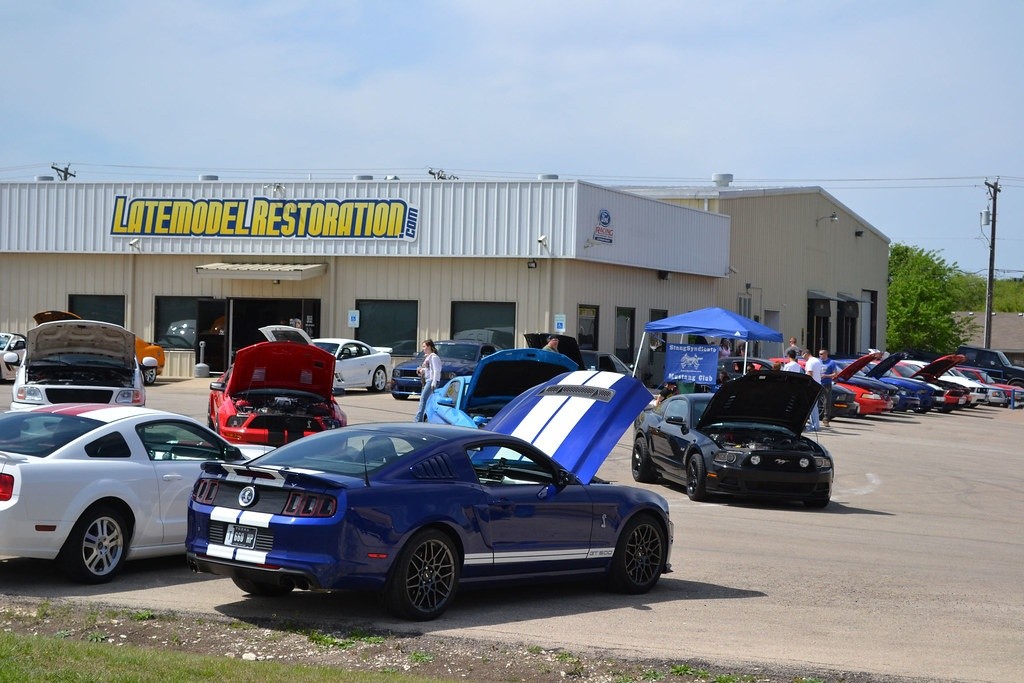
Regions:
[{"x1": 954, "y1": 344, "x2": 1024, "y2": 387}]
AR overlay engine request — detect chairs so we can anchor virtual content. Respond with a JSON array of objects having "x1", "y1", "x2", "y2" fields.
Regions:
[
  {"x1": 677, "y1": 404, "x2": 701, "y2": 427},
  {"x1": 52, "y1": 416, "x2": 83, "y2": 450},
  {"x1": 12, "y1": 341, "x2": 26, "y2": 350},
  {"x1": 439, "y1": 346, "x2": 449, "y2": 356},
  {"x1": 97, "y1": 436, "x2": 130, "y2": 456},
  {"x1": 355, "y1": 436, "x2": 399, "y2": 464},
  {"x1": 338, "y1": 348, "x2": 352, "y2": 359},
  {"x1": 410, "y1": 453, "x2": 458, "y2": 478}
]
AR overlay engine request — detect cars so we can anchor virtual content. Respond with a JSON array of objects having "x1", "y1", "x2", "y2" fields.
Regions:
[
  {"x1": 391, "y1": 340, "x2": 501, "y2": 400},
  {"x1": 0, "y1": 332, "x2": 28, "y2": 384},
  {"x1": 0, "y1": 404, "x2": 251, "y2": 586},
  {"x1": 704, "y1": 358, "x2": 859, "y2": 421},
  {"x1": 167, "y1": 320, "x2": 197, "y2": 347},
  {"x1": 208, "y1": 341, "x2": 348, "y2": 448},
  {"x1": 424, "y1": 349, "x2": 582, "y2": 429},
  {"x1": 521, "y1": 333, "x2": 627, "y2": 373},
  {"x1": 258, "y1": 324, "x2": 394, "y2": 395},
  {"x1": 185, "y1": 371, "x2": 674, "y2": 620},
  {"x1": 33, "y1": 310, "x2": 166, "y2": 387},
  {"x1": 768, "y1": 353, "x2": 1024, "y2": 418},
  {"x1": 630, "y1": 368, "x2": 834, "y2": 509}
]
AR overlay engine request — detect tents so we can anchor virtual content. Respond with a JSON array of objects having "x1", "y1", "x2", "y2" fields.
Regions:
[{"x1": 632, "y1": 305, "x2": 785, "y2": 377}]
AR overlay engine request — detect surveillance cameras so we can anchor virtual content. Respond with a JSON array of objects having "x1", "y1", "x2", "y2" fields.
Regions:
[
  {"x1": 538, "y1": 236, "x2": 547, "y2": 242},
  {"x1": 130, "y1": 239, "x2": 139, "y2": 246}
]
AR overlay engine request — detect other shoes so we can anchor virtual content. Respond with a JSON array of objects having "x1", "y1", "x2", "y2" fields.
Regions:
[
  {"x1": 807, "y1": 427, "x2": 820, "y2": 432},
  {"x1": 821, "y1": 420, "x2": 829, "y2": 427}
]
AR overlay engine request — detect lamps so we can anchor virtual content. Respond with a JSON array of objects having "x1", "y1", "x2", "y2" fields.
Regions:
[
  {"x1": 262, "y1": 183, "x2": 286, "y2": 192},
  {"x1": 527, "y1": 259, "x2": 537, "y2": 269},
  {"x1": 273, "y1": 280, "x2": 280, "y2": 284},
  {"x1": 855, "y1": 230, "x2": 865, "y2": 240},
  {"x1": 815, "y1": 212, "x2": 840, "y2": 226}
]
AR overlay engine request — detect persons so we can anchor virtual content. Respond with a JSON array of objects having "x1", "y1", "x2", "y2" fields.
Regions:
[
  {"x1": 719, "y1": 338, "x2": 745, "y2": 357},
  {"x1": 541, "y1": 335, "x2": 559, "y2": 353},
  {"x1": 773, "y1": 360, "x2": 781, "y2": 371},
  {"x1": 819, "y1": 348, "x2": 838, "y2": 427},
  {"x1": 802, "y1": 349, "x2": 822, "y2": 432},
  {"x1": 784, "y1": 337, "x2": 804, "y2": 357},
  {"x1": 656, "y1": 382, "x2": 679, "y2": 406},
  {"x1": 415, "y1": 340, "x2": 442, "y2": 422},
  {"x1": 746, "y1": 363, "x2": 755, "y2": 372},
  {"x1": 783, "y1": 350, "x2": 804, "y2": 374}
]
